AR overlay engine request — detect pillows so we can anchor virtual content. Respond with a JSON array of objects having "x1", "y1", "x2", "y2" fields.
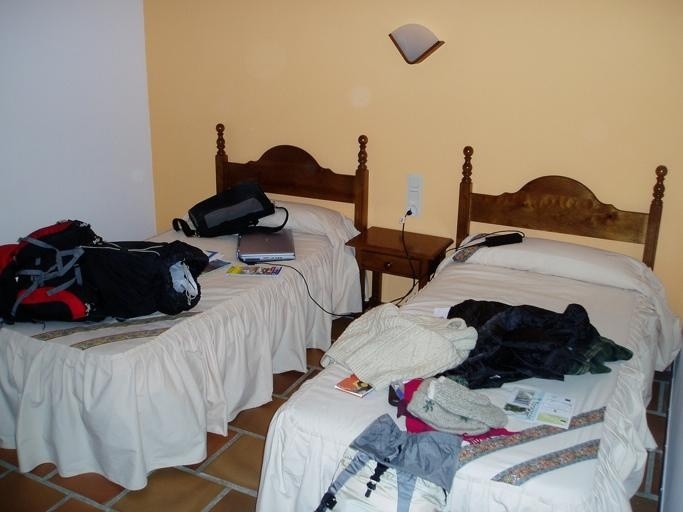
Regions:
[
  {"x1": 463, "y1": 232, "x2": 662, "y2": 294},
  {"x1": 256, "y1": 200, "x2": 360, "y2": 248}
]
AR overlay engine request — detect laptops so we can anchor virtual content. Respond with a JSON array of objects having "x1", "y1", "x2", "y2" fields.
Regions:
[{"x1": 235, "y1": 226, "x2": 296, "y2": 263}]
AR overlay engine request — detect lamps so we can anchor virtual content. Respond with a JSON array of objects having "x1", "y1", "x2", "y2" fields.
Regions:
[{"x1": 388, "y1": 24, "x2": 444, "y2": 64}]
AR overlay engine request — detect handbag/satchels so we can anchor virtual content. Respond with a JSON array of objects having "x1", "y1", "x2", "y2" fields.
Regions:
[
  {"x1": 78, "y1": 238, "x2": 209, "y2": 319},
  {"x1": 172, "y1": 181, "x2": 290, "y2": 239}
]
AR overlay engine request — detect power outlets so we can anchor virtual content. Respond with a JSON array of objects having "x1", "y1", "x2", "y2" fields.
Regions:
[{"x1": 406, "y1": 202, "x2": 420, "y2": 217}]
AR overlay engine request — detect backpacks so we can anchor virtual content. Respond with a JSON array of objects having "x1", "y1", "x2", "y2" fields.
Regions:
[{"x1": 0, "y1": 219, "x2": 102, "y2": 324}]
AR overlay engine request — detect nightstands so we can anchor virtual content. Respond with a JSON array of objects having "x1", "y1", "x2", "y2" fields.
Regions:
[{"x1": 347, "y1": 226, "x2": 454, "y2": 309}]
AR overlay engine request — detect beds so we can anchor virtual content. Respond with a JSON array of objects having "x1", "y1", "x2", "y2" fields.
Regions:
[
  {"x1": 0, "y1": 123, "x2": 367, "y2": 491},
  {"x1": 252, "y1": 147, "x2": 668, "y2": 512}
]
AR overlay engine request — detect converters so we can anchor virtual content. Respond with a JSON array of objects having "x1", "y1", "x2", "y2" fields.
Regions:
[{"x1": 485, "y1": 233, "x2": 524, "y2": 247}]
